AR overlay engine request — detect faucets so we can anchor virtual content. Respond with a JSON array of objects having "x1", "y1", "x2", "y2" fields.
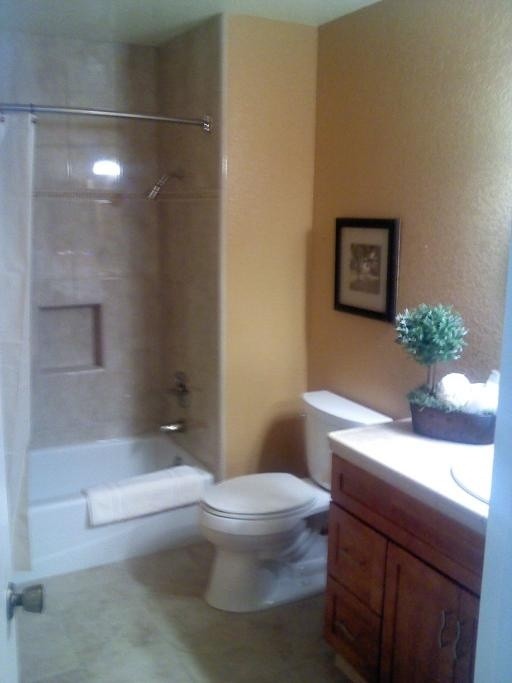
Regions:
[{"x1": 159, "y1": 418, "x2": 186, "y2": 433}]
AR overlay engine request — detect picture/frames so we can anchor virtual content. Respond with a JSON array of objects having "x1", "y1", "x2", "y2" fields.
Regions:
[{"x1": 333, "y1": 218, "x2": 400, "y2": 329}]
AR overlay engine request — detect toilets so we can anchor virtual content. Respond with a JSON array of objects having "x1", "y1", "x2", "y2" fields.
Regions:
[{"x1": 196, "y1": 388, "x2": 395, "y2": 614}]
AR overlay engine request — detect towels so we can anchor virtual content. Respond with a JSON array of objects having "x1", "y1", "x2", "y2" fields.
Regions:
[{"x1": 82, "y1": 466, "x2": 215, "y2": 531}]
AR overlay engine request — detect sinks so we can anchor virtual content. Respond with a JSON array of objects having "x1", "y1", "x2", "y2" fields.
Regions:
[{"x1": 450, "y1": 443, "x2": 495, "y2": 505}]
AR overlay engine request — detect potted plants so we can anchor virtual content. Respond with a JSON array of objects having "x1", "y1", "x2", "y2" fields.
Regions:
[{"x1": 392, "y1": 305, "x2": 497, "y2": 444}]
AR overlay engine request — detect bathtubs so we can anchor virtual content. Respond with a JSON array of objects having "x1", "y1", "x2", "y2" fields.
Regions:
[{"x1": 15, "y1": 430, "x2": 215, "y2": 587}]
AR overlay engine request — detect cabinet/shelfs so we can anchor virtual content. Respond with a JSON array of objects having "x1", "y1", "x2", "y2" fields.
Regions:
[{"x1": 325, "y1": 452, "x2": 486, "y2": 681}]
